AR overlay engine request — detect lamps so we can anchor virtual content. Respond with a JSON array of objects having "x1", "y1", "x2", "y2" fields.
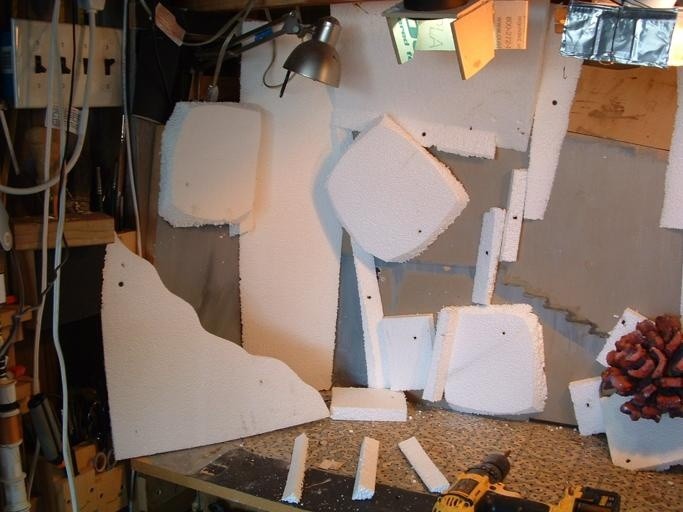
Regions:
[{"x1": 223, "y1": 10, "x2": 342, "y2": 98}]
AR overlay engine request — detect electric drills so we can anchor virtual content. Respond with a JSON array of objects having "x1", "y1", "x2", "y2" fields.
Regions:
[{"x1": 433, "y1": 450, "x2": 621, "y2": 512}]
